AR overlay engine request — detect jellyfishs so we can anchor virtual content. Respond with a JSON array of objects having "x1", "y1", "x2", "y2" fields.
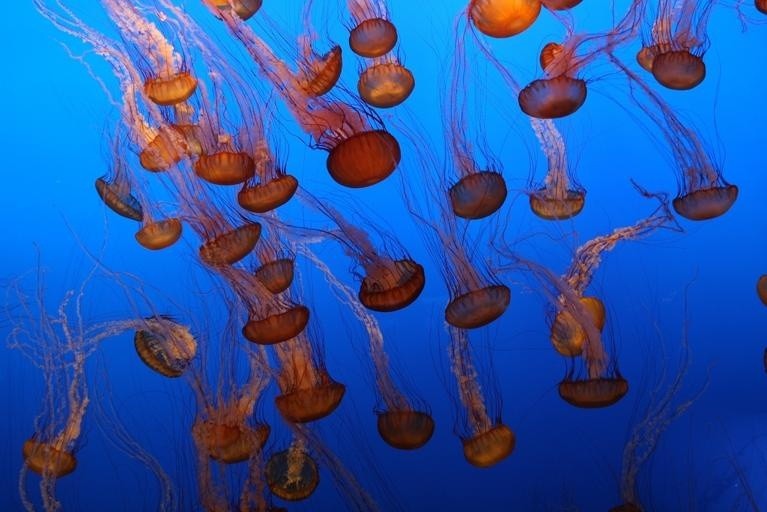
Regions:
[{"x1": 0, "y1": 0, "x2": 766, "y2": 511}]
